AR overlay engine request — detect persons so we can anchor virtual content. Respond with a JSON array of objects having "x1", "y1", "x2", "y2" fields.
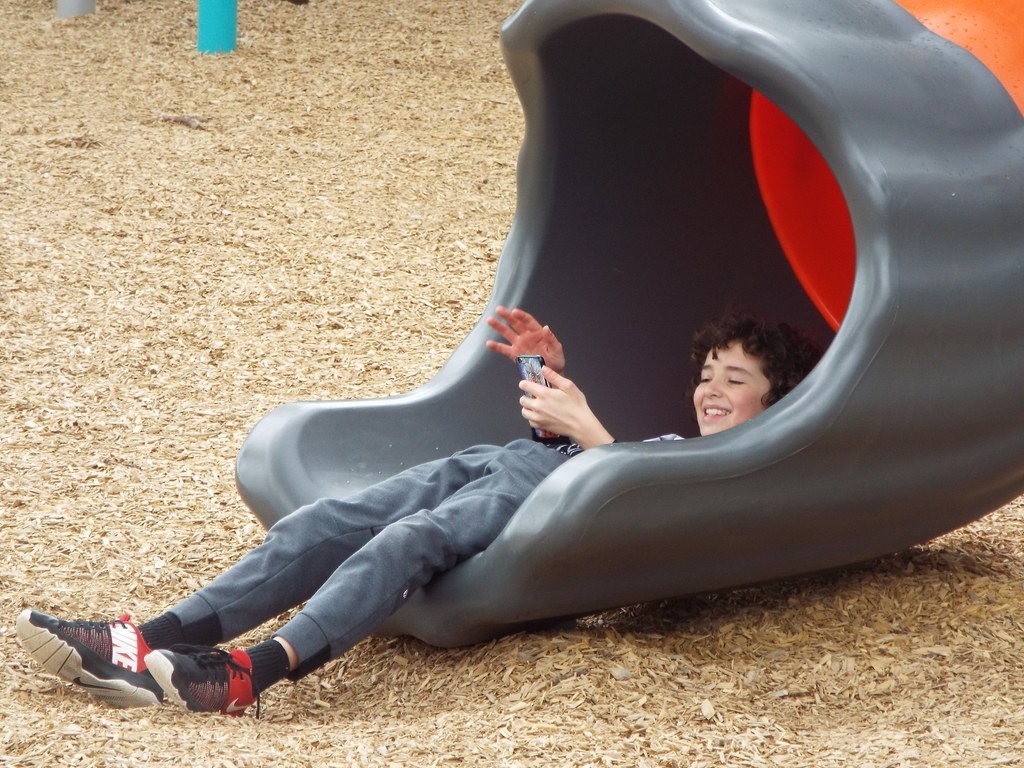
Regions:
[{"x1": 17, "y1": 303, "x2": 828, "y2": 717}]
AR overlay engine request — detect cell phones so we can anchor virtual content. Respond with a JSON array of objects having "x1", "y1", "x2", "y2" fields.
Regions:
[{"x1": 513, "y1": 354, "x2": 563, "y2": 442}]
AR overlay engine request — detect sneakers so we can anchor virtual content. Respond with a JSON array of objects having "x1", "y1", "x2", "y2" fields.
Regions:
[
  {"x1": 15, "y1": 609, "x2": 163, "y2": 709},
  {"x1": 143, "y1": 643, "x2": 260, "y2": 719}
]
